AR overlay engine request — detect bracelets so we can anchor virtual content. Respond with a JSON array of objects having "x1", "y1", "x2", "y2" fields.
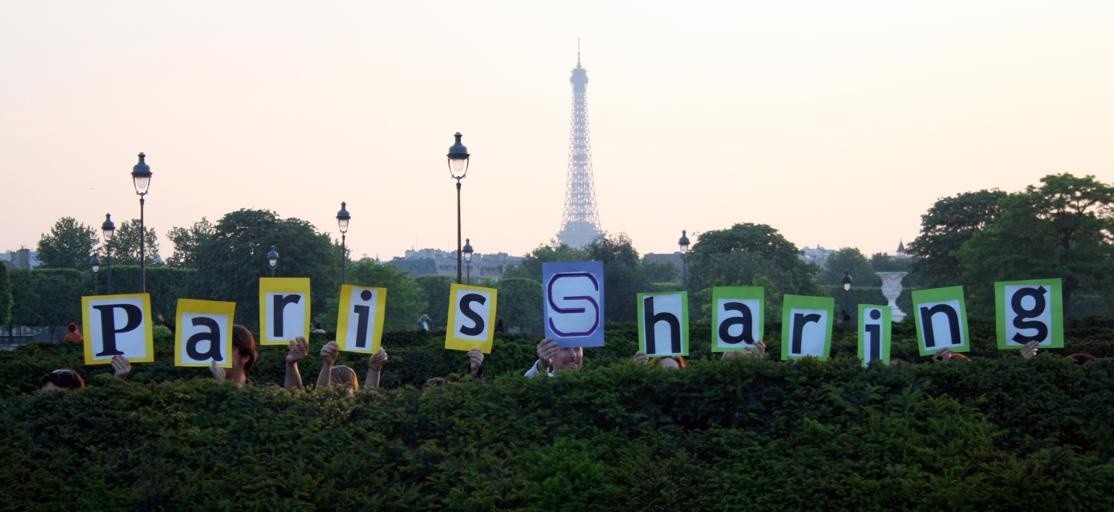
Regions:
[
  {"x1": 369, "y1": 367, "x2": 380, "y2": 371},
  {"x1": 427, "y1": 319, "x2": 430, "y2": 322}
]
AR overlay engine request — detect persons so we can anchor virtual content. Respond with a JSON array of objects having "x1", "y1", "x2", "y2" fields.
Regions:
[
  {"x1": 64, "y1": 322, "x2": 84, "y2": 342},
  {"x1": 633, "y1": 351, "x2": 686, "y2": 368},
  {"x1": 932, "y1": 347, "x2": 971, "y2": 363},
  {"x1": 111, "y1": 354, "x2": 132, "y2": 380},
  {"x1": 523, "y1": 337, "x2": 583, "y2": 378},
  {"x1": 315, "y1": 341, "x2": 387, "y2": 400},
  {"x1": 422, "y1": 348, "x2": 484, "y2": 391},
  {"x1": 207, "y1": 324, "x2": 308, "y2": 391},
  {"x1": 1020, "y1": 340, "x2": 1039, "y2": 360},
  {"x1": 720, "y1": 340, "x2": 766, "y2": 362},
  {"x1": 416, "y1": 311, "x2": 432, "y2": 331}
]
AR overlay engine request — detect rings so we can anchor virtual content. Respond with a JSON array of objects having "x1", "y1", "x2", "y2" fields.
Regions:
[
  {"x1": 937, "y1": 356, "x2": 942, "y2": 361},
  {"x1": 119, "y1": 367, "x2": 121, "y2": 369},
  {"x1": 547, "y1": 350, "x2": 551, "y2": 353}
]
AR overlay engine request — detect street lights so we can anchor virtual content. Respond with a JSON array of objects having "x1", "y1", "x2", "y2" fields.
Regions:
[
  {"x1": 265, "y1": 243, "x2": 279, "y2": 276},
  {"x1": 101, "y1": 212, "x2": 116, "y2": 292},
  {"x1": 840, "y1": 271, "x2": 852, "y2": 323},
  {"x1": 677, "y1": 229, "x2": 691, "y2": 291},
  {"x1": 128, "y1": 152, "x2": 153, "y2": 293},
  {"x1": 445, "y1": 130, "x2": 470, "y2": 284},
  {"x1": 460, "y1": 237, "x2": 473, "y2": 286},
  {"x1": 334, "y1": 200, "x2": 351, "y2": 284},
  {"x1": 90, "y1": 253, "x2": 99, "y2": 294}
]
